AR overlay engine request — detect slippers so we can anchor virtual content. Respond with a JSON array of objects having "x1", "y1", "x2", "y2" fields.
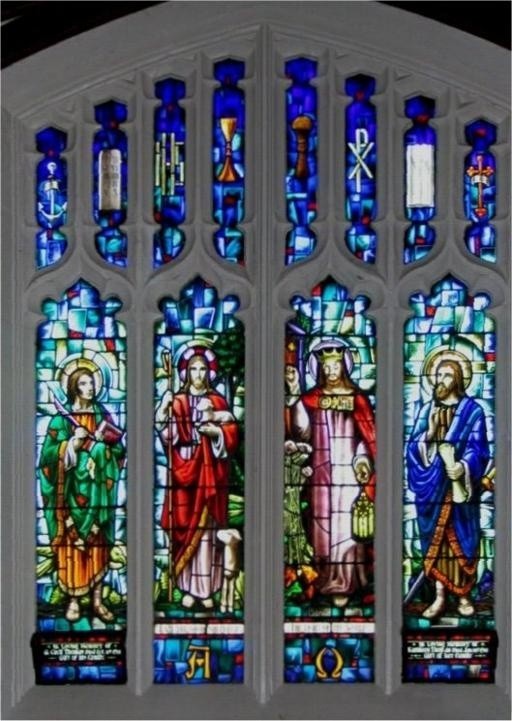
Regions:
[
  {"x1": 65, "y1": 603, "x2": 116, "y2": 624},
  {"x1": 422, "y1": 597, "x2": 474, "y2": 618}
]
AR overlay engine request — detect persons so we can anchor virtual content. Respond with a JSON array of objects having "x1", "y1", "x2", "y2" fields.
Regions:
[
  {"x1": 407, "y1": 359, "x2": 491, "y2": 617},
  {"x1": 154, "y1": 353, "x2": 239, "y2": 608},
  {"x1": 36, "y1": 367, "x2": 125, "y2": 624},
  {"x1": 285, "y1": 355, "x2": 376, "y2": 608}
]
